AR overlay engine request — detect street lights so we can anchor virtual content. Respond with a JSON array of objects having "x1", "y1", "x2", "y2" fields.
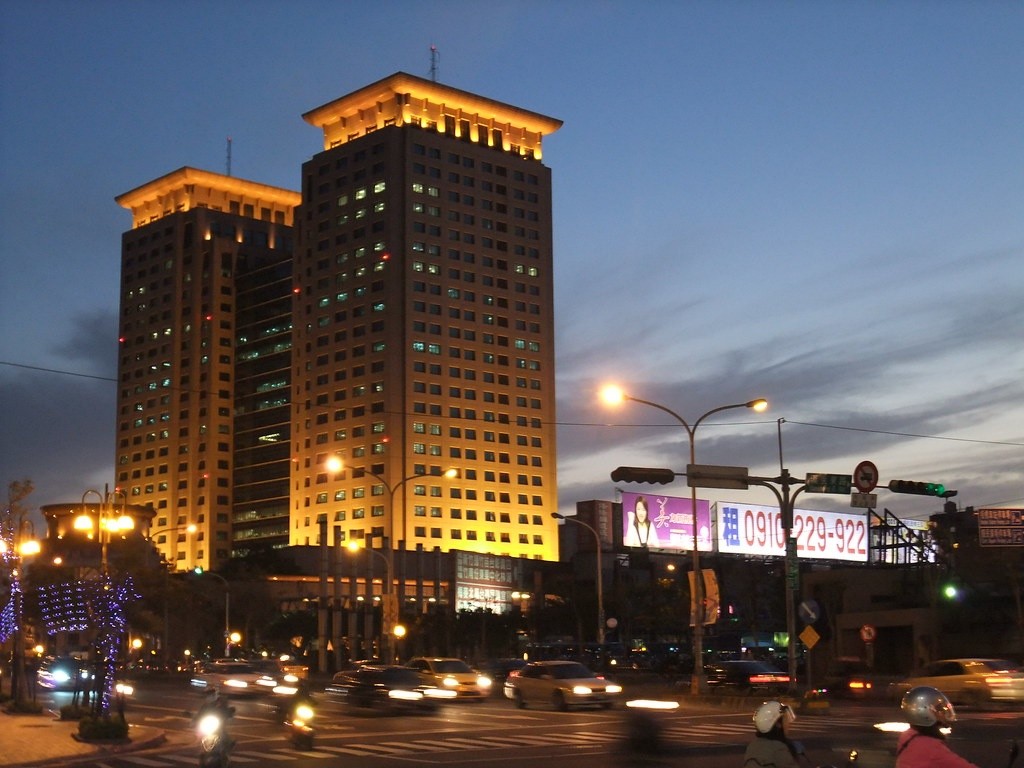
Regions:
[
  {"x1": 328, "y1": 459, "x2": 454, "y2": 666},
  {"x1": 195, "y1": 565, "x2": 229, "y2": 659},
  {"x1": 602, "y1": 383, "x2": 768, "y2": 695},
  {"x1": 551, "y1": 512, "x2": 602, "y2": 645},
  {"x1": 72, "y1": 482, "x2": 136, "y2": 720},
  {"x1": 0, "y1": 515, "x2": 41, "y2": 699}
]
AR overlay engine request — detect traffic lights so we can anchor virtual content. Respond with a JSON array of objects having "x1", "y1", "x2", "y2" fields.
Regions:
[
  {"x1": 889, "y1": 480, "x2": 945, "y2": 496},
  {"x1": 611, "y1": 466, "x2": 675, "y2": 485},
  {"x1": 939, "y1": 581, "x2": 957, "y2": 610}
]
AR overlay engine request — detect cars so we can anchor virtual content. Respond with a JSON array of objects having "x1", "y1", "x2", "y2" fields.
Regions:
[
  {"x1": 888, "y1": 656, "x2": 1024, "y2": 711},
  {"x1": 503, "y1": 660, "x2": 623, "y2": 712},
  {"x1": 323, "y1": 656, "x2": 493, "y2": 716},
  {"x1": 32, "y1": 654, "x2": 309, "y2": 700}
]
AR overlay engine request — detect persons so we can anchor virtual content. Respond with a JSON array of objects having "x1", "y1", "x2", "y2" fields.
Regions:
[
  {"x1": 192, "y1": 686, "x2": 227, "y2": 721},
  {"x1": 628, "y1": 496, "x2": 659, "y2": 546},
  {"x1": 743, "y1": 701, "x2": 804, "y2": 768},
  {"x1": 280, "y1": 681, "x2": 316, "y2": 720},
  {"x1": 894, "y1": 687, "x2": 978, "y2": 768}
]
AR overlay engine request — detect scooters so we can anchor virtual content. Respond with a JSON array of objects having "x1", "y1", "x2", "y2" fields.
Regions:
[{"x1": 182, "y1": 705, "x2": 237, "y2": 768}]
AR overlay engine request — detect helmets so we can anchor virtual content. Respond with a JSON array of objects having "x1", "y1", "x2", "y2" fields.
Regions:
[
  {"x1": 900, "y1": 687, "x2": 956, "y2": 728},
  {"x1": 753, "y1": 701, "x2": 783, "y2": 734}
]
